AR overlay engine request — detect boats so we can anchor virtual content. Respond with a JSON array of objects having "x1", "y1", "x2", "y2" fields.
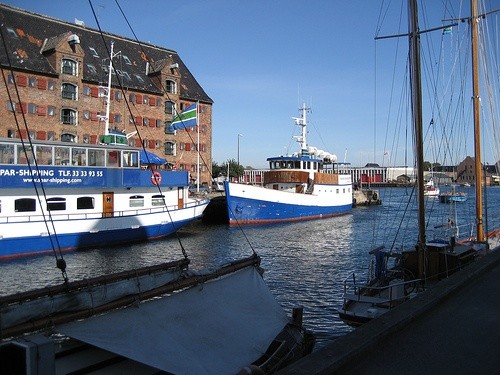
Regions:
[
  {"x1": 438, "y1": 191, "x2": 469, "y2": 203},
  {"x1": 0, "y1": 125, "x2": 212, "y2": 261},
  {"x1": 421, "y1": 182, "x2": 439, "y2": 196},
  {"x1": 221, "y1": 81, "x2": 355, "y2": 227}
]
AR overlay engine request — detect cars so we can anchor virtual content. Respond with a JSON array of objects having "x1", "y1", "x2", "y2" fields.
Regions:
[
  {"x1": 212, "y1": 180, "x2": 224, "y2": 192},
  {"x1": 187, "y1": 181, "x2": 211, "y2": 195}
]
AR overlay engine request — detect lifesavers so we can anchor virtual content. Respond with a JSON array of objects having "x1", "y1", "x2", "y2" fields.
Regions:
[{"x1": 151, "y1": 172, "x2": 162, "y2": 185}]
